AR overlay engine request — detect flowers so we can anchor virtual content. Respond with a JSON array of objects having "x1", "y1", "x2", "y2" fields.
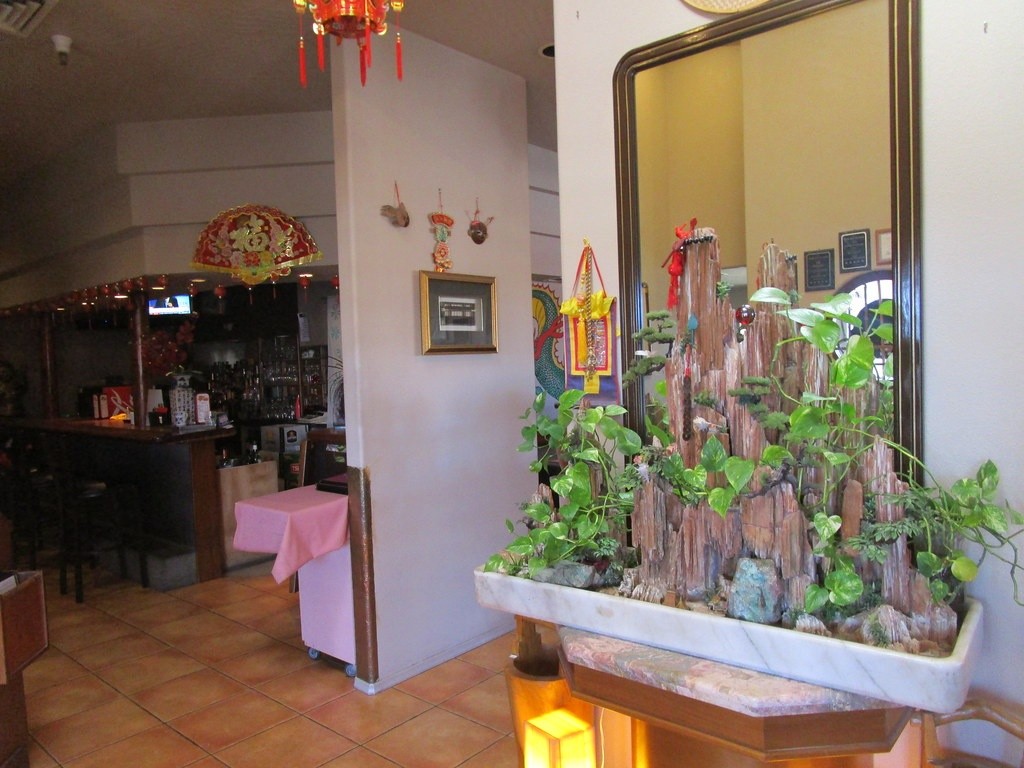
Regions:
[{"x1": 130, "y1": 310, "x2": 203, "y2": 376}]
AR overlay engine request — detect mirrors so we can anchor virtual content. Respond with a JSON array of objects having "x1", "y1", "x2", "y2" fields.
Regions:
[{"x1": 612, "y1": 0, "x2": 924, "y2": 572}]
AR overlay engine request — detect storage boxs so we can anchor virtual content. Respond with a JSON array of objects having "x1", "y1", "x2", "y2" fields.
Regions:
[
  {"x1": 218, "y1": 461, "x2": 278, "y2": 571},
  {"x1": 260, "y1": 424, "x2": 309, "y2": 479}
]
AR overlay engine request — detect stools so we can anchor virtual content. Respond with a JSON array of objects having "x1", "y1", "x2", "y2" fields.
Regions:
[{"x1": 9, "y1": 434, "x2": 149, "y2": 604}]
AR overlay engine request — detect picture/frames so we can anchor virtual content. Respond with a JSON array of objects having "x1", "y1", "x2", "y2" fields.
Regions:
[{"x1": 419, "y1": 269, "x2": 500, "y2": 354}]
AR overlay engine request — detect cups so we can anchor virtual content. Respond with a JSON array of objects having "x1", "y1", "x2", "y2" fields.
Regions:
[{"x1": 174, "y1": 412, "x2": 188, "y2": 427}]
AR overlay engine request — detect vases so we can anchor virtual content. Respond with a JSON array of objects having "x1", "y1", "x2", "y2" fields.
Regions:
[{"x1": 169, "y1": 375, "x2": 197, "y2": 425}]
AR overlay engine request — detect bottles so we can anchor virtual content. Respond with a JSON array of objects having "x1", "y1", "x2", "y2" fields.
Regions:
[{"x1": 218, "y1": 448, "x2": 231, "y2": 468}]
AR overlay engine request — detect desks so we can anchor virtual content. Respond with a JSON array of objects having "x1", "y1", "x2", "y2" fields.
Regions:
[{"x1": 555, "y1": 623, "x2": 915, "y2": 768}]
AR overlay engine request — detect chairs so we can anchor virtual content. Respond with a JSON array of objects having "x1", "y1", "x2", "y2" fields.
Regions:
[{"x1": 288, "y1": 439, "x2": 311, "y2": 595}]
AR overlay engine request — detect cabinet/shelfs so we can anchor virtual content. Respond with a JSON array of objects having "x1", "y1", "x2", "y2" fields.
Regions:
[
  {"x1": 311, "y1": 428, "x2": 346, "y2": 486},
  {"x1": 199, "y1": 331, "x2": 328, "y2": 457},
  {"x1": 232, "y1": 482, "x2": 357, "y2": 678}
]
